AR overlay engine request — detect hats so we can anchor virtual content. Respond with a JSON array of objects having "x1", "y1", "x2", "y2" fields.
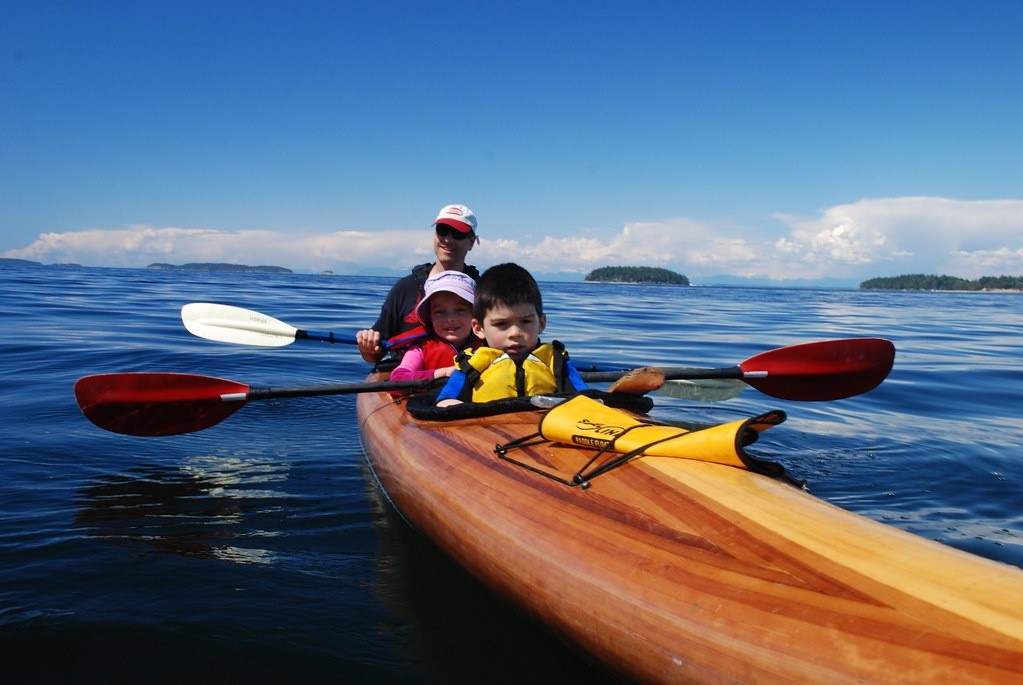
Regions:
[
  {"x1": 431, "y1": 204, "x2": 478, "y2": 234},
  {"x1": 416, "y1": 270, "x2": 477, "y2": 325}
]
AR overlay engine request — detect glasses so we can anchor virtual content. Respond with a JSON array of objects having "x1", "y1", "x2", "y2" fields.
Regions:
[{"x1": 436, "y1": 224, "x2": 470, "y2": 240}]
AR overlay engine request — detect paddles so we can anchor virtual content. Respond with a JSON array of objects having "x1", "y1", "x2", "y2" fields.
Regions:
[
  {"x1": 180, "y1": 303, "x2": 747, "y2": 402},
  {"x1": 75, "y1": 336, "x2": 896, "y2": 437}
]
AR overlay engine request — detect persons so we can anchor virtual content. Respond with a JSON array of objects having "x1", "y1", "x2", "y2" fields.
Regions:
[{"x1": 355, "y1": 205, "x2": 587, "y2": 408}]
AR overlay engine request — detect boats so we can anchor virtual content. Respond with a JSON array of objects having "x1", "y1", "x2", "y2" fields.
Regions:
[{"x1": 355, "y1": 356, "x2": 1023, "y2": 685}]
